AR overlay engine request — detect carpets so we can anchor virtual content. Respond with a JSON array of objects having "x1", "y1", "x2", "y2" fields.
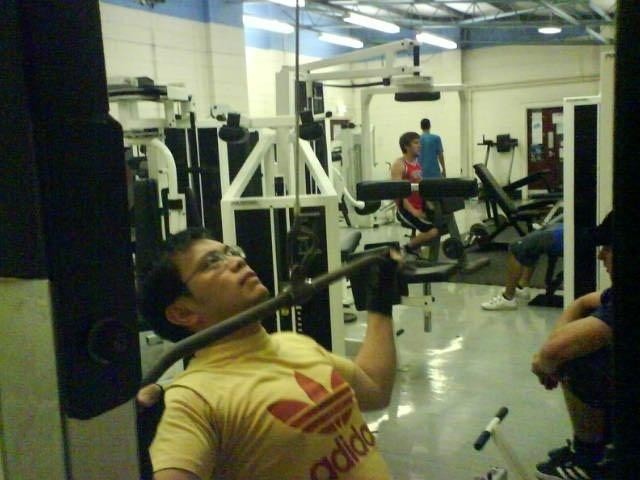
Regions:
[{"x1": 423, "y1": 222, "x2": 564, "y2": 290}]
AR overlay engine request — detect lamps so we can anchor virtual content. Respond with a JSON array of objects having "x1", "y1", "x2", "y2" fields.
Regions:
[{"x1": 241, "y1": 0, "x2": 459, "y2": 49}]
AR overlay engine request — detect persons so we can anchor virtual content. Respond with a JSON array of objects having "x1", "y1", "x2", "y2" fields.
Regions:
[
  {"x1": 531, "y1": 211, "x2": 615, "y2": 480},
  {"x1": 480, "y1": 221, "x2": 562, "y2": 311},
  {"x1": 416, "y1": 119, "x2": 446, "y2": 178},
  {"x1": 136, "y1": 226, "x2": 407, "y2": 480},
  {"x1": 391, "y1": 132, "x2": 439, "y2": 259}
]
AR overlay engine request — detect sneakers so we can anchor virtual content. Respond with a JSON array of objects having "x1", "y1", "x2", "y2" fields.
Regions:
[
  {"x1": 481, "y1": 286, "x2": 531, "y2": 310},
  {"x1": 536, "y1": 439, "x2": 615, "y2": 480}
]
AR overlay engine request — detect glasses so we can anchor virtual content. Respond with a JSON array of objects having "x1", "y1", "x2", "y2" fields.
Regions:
[{"x1": 184, "y1": 245, "x2": 247, "y2": 285}]
